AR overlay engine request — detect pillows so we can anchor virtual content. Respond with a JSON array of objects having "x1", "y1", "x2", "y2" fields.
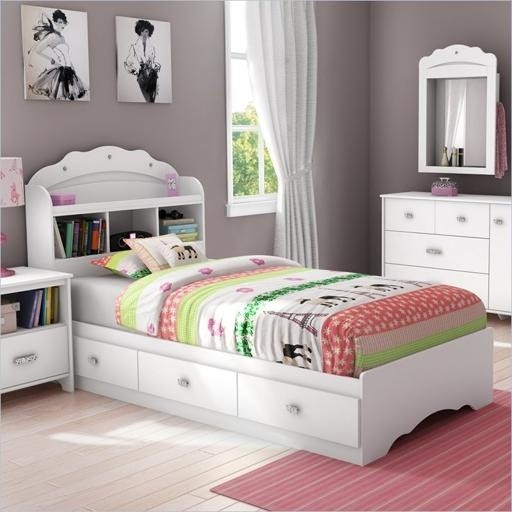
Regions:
[
  {"x1": 87, "y1": 249, "x2": 151, "y2": 281},
  {"x1": 158, "y1": 242, "x2": 210, "y2": 268},
  {"x1": 122, "y1": 233, "x2": 183, "y2": 273}
]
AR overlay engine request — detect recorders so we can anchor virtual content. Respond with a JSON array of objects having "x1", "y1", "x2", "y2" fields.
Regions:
[{"x1": 110, "y1": 231, "x2": 152, "y2": 252}]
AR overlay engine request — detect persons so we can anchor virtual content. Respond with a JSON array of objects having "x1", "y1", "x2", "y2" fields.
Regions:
[
  {"x1": 26, "y1": 9, "x2": 88, "y2": 101},
  {"x1": 124, "y1": 20, "x2": 161, "y2": 102}
]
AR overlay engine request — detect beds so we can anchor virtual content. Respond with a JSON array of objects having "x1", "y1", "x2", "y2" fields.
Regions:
[{"x1": 24, "y1": 145, "x2": 494, "y2": 468}]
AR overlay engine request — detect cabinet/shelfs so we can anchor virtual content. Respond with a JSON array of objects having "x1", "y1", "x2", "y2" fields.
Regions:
[
  {"x1": 380, "y1": 191, "x2": 512, "y2": 320},
  {"x1": 0, "y1": 267, "x2": 74, "y2": 393}
]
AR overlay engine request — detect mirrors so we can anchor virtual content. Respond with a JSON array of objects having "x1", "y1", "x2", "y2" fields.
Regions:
[{"x1": 417, "y1": 44, "x2": 501, "y2": 176}]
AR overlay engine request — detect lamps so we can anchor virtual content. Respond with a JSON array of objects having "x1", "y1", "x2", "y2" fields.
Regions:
[{"x1": 0, "y1": 157, "x2": 25, "y2": 277}]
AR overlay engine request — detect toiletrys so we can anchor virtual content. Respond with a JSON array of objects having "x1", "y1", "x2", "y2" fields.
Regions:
[
  {"x1": 452, "y1": 147, "x2": 457, "y2": 166},
  {"x1": 458, "y1": 148, "x2": 464, "y2": 166},
  {"x1": 440, "y1": 146, "x2": 448, "y2": 166},
  {"x1": 441, "y1": 145, "x2": 466, "y2": 166}
]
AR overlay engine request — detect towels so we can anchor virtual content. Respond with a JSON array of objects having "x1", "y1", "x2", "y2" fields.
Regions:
[{"x1": 496, "y1": 102, "x2": 507, "y2": 178}]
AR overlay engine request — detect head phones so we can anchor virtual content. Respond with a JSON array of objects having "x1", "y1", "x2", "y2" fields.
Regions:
[{"x1": 159, "y1": 209, "x2": 183, "y2": 219}]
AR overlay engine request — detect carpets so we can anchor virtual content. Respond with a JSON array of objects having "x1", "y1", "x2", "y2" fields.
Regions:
[{"x1": 209, "y1": 388, "x2": 512, "y2": 512}]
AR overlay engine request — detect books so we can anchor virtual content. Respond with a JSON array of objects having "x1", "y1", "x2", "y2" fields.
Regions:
[
  {"x1": 16, "y1": 287, "x2": 60, "y2": 328},
  {"x1": 53, "y1": 215, "x2": 106, "y2": 257},
  {"x1": 159, "y1": 217, "x2": 200, "y2": 242}
]
